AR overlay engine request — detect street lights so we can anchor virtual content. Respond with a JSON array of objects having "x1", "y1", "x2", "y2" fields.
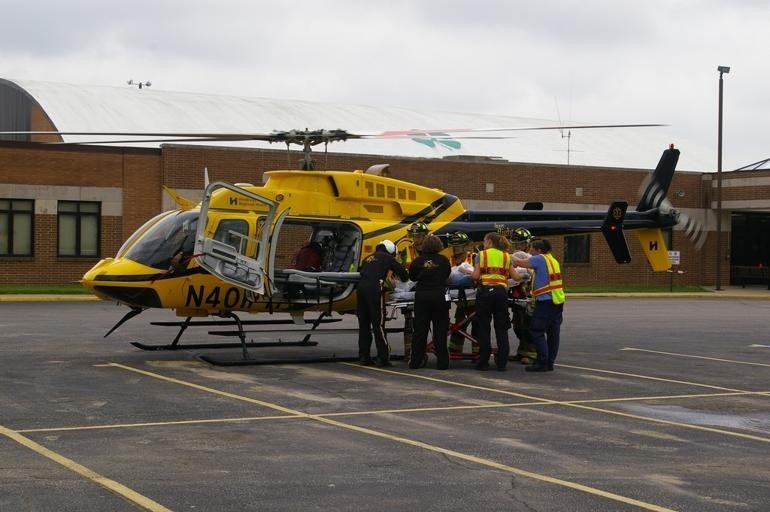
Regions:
[{"x1": 716, "y1": 66, "x2": 730, "y2": 288}]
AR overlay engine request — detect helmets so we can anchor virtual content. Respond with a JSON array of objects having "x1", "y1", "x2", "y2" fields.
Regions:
[
  {"x1": 510, "y1": 227, "x2": 535, "y2": 243},
  {"x1": 315, "y1": 230, "x2": 333, "y2": 246},
  {"x1": 407, "y1": 222, "x2": 430, "y2": 237},
  {"x1": 448, "y1": 231, "x2": 469, "y2": 246},
  {"x1": 377, "y1": 240, "x2": 398, "y2": 256}
]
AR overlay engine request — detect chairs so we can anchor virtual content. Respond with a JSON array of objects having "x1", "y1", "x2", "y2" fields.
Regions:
[{"x1": 275, "y1": 229, "x2": 356, "y2": 296}]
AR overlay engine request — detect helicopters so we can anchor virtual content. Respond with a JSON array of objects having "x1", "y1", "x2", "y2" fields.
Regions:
[{"x1": 0, "y1": 123, "x2": 705, "y2": 367}]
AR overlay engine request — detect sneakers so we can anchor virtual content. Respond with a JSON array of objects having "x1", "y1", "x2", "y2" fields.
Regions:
[{"x1": 519, "y1": 355, "x2": 554, "y2": 371}]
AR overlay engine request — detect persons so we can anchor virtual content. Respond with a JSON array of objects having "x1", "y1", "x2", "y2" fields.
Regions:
[
  {"x1": 291, "y1": 239, "x2": 321, "y2": 271},
  {"x1": 353, "y1": 222, "x2": 567, "y2": 372}
]
